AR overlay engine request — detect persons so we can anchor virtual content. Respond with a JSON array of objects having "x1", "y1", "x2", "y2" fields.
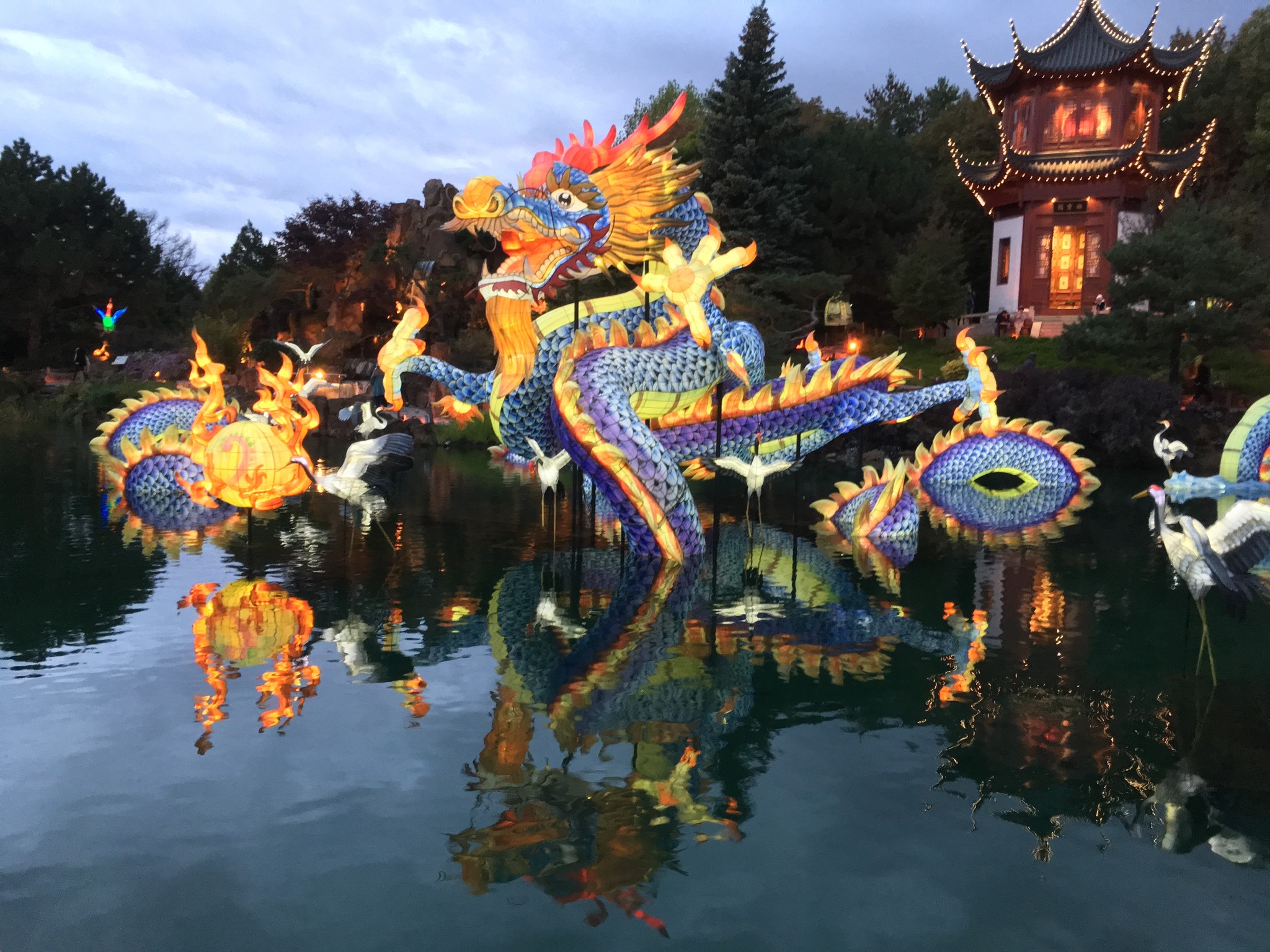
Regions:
[
  {"x1": 1170, "y1": 333, "x2": 1212, "y2": 404},
  {"x1": 995, "y1": 310, "x2": 1010, "y2": 337},
  {"x1": 1091, "y1": 294, "x2": 1106, "y2": 318},
  {"x1": 1013, "y1": 303, "x2": 1027, "y2": 340},
  {"x1": 69, "y1": 347, "x2": 89, "y2": 382}
]
